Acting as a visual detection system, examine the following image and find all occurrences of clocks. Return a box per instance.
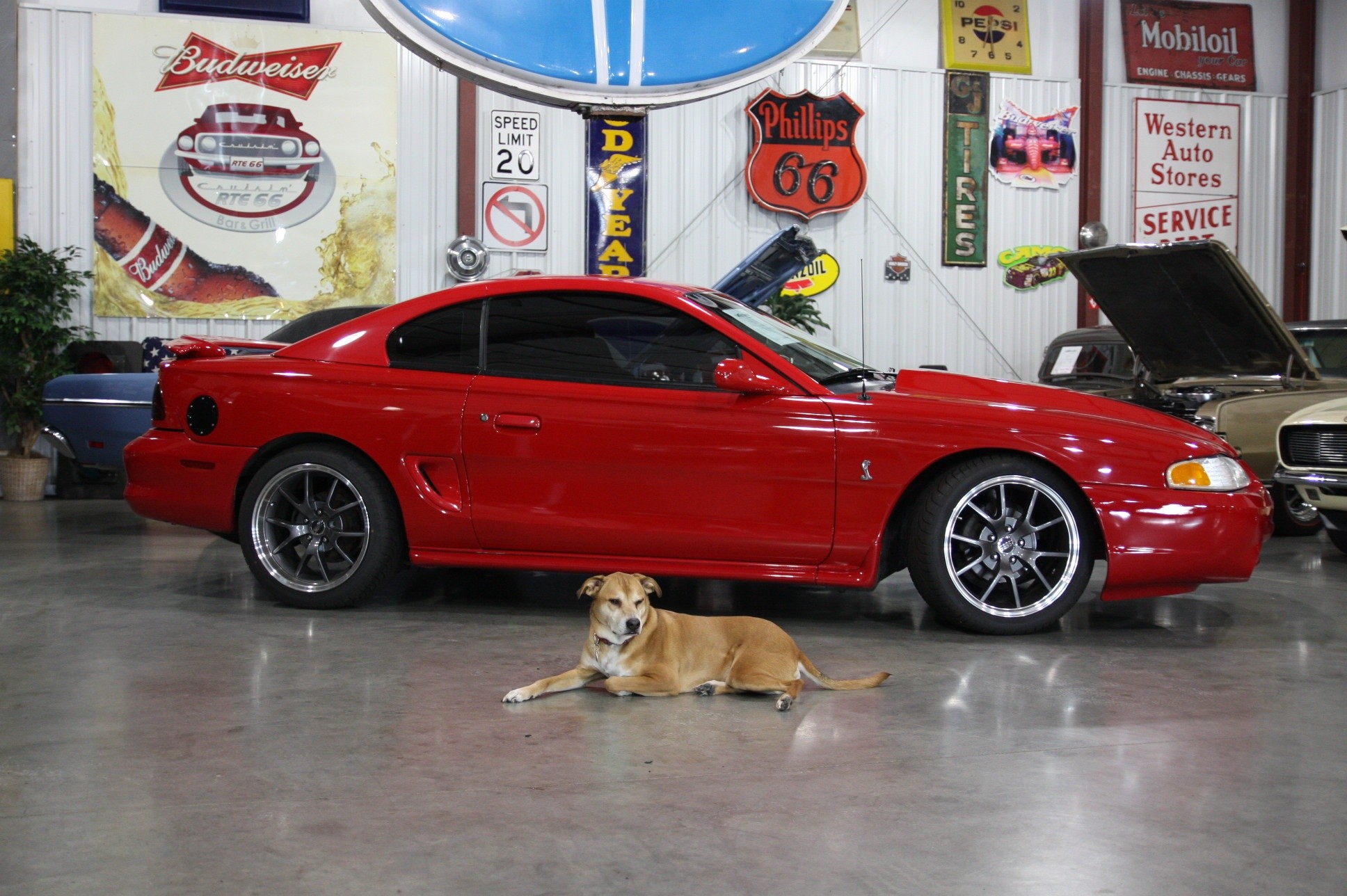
[942,0,1033,76]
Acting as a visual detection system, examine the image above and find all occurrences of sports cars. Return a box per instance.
[1002,256,1068,289]
[124,278,1271,634]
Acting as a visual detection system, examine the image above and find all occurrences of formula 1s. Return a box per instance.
[990,122,1076,175]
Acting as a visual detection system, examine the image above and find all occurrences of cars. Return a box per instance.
[1053,241,1347,539]
[1276,393,1347,556]
[32,304,402,496]
[176,104,323,182]
[1030,327,1162,404]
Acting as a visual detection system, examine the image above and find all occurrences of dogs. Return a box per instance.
[501,570,892,714]
